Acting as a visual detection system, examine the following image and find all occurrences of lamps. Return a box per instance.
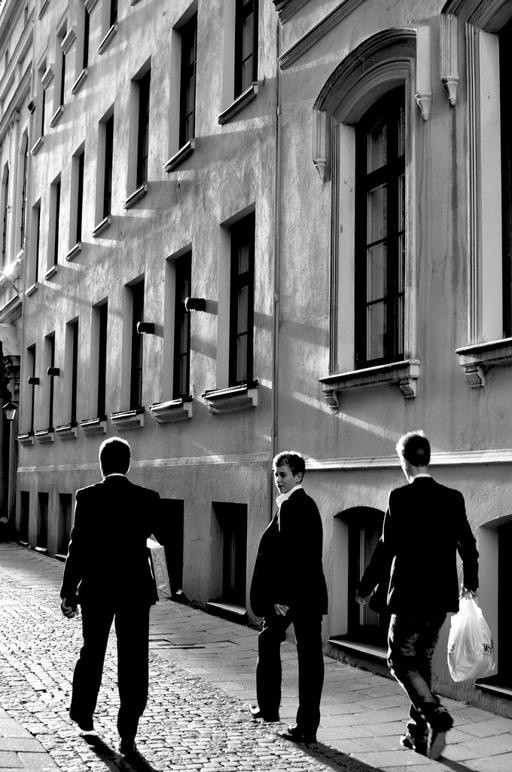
[185,297,206,311]
[48,367,59,376]
[28,377,39,384]
[137,322,155,333]
[2,401,18,421]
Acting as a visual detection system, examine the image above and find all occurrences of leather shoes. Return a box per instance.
[286,722,318,743]
[243,703,280,722]
[118,737,139,755]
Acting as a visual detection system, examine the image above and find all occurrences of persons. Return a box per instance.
[59,436,173,754]
[354,433,480,758]
[248,450,329,744]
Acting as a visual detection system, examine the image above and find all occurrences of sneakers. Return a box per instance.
[400,706,454,760]
